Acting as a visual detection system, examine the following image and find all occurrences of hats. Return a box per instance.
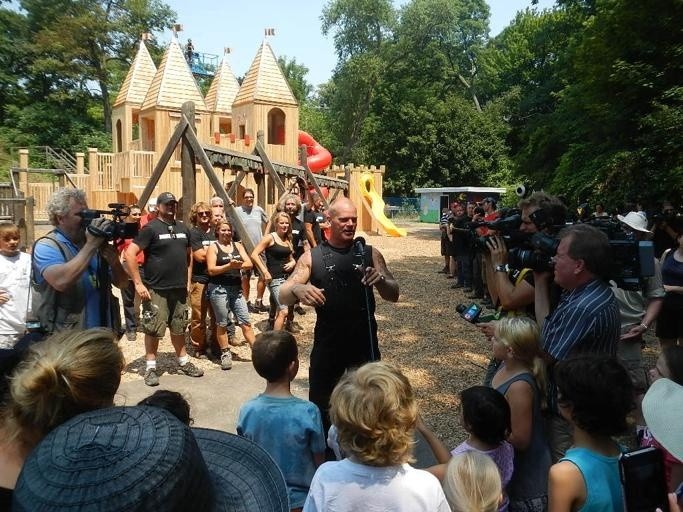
[641,377,683,462]
[616,210,653,236]
[156,192,180,207]
[8,405,292,511]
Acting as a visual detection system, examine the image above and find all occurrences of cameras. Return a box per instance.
[617,446,670,512]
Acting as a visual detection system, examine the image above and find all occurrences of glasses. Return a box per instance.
[198,211,211,219]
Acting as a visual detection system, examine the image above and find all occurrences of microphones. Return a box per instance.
[456,302,484,324]
[354,236,366,264]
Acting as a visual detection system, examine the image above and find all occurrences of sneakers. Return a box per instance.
[255,303,270,312]
[437,268,448,274]
[479,299,493,303]
[463,288,473,293]
[485,302,495,310]
[294,305,319,317]
[220,351,235,372]
[175,360,205,379]
[464,290,477,298]
[450,282,463,290]
[144,368,160,386]
[246,302,260,313]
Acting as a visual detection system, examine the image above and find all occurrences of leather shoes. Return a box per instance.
[126,327,141,342]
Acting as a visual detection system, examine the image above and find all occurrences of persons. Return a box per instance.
[235,328,326,512]
[264,193,306,317]
[326,414,346,460]
[210,196,225,212]
[443,449,504,512]
[302,361,454,512]
[303,193,325,248]
[541,224,622,464]
[29,184,129,335]
[421,386,514,511]
[278,198,399,460]
[206,219,257,371]
[639,343,682,494]
[231,188,271,313]
[482,317,553,511]
[0,324,127,512]
[124,191,204,387]
[137,389,195,429]
[114,204,149,342]
[0,221,40,400]
[570,192,682,228]
[439,192,570,325]
[656,229,683,385]
[642,378,683,511]
[250,211,298,330]
[187,201,218,359]
[611,211,667,394]
[546,350,636,511]
[211,208,224,240]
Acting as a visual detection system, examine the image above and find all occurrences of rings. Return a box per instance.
[380,276,384,280]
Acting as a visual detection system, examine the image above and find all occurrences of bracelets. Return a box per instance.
[291,285,298,300]
[641,324,649,329]
